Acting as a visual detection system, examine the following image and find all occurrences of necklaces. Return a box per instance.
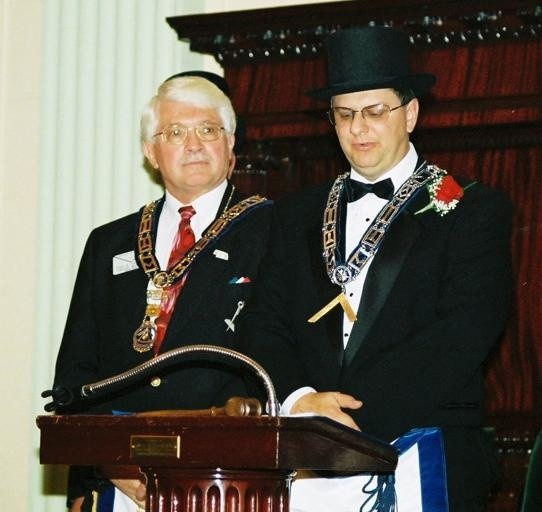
[134,194,267,353]
[307,164,444,322]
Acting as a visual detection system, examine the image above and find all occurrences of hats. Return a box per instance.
[303,26,436,101]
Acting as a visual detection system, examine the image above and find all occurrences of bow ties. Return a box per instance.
[343,178,395,202]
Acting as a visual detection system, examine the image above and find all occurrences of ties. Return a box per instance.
[151,205,197,357]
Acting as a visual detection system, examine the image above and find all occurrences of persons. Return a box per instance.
[226,30,515,511]
[49,75,295,511]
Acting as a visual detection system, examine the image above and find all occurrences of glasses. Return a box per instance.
[153,119,225,145]
[326,103,405,126]
